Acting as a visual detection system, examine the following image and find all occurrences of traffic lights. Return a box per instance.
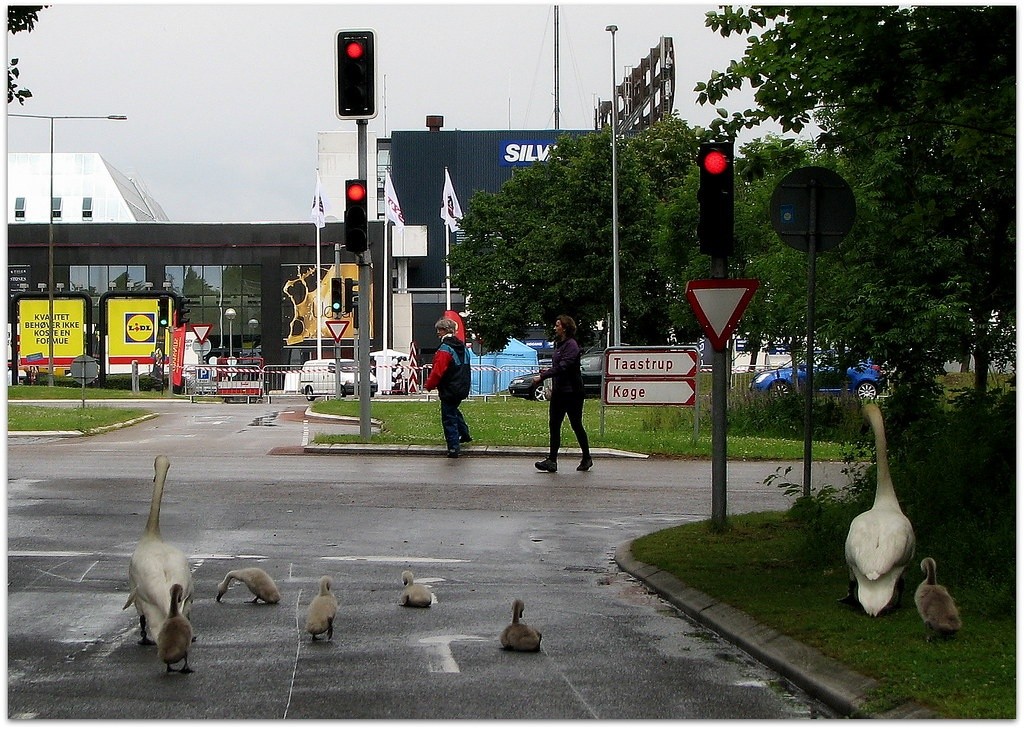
[334,29,378,119]
[344,179,368,253]
[696,141,734,257]
[331,278,341,312]
[345,278,358,312]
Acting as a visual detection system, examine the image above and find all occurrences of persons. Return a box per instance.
[426,318,472,459]
[533,316,594,472]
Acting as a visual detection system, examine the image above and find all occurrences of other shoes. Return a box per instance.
[447,448,458,458]
[575,456,594,471]
[535,456,558,472]
[459,434,472,443]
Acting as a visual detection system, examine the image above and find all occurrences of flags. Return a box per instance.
[311,172,325,228]
[440,170,463,233]
[385,172,404,226]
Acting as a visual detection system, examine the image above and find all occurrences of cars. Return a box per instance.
[509,351,603,402]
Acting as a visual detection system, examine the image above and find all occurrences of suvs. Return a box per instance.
[748,350,885,402]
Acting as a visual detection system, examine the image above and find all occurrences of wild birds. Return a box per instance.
[122,455,194,648]
[844,404,916,618]
[215,567,282,606]
[306,576,338,644]
[500,600,543,652]
[157,584,193,673]
[914,557,963,643]
[399,571,434,607]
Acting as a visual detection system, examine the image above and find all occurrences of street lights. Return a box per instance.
[8,114,128,386]
[605,24,623,382]
[247,319,259,367]
[225,308,237,357]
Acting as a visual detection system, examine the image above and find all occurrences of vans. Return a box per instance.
[300,358,378,402]
